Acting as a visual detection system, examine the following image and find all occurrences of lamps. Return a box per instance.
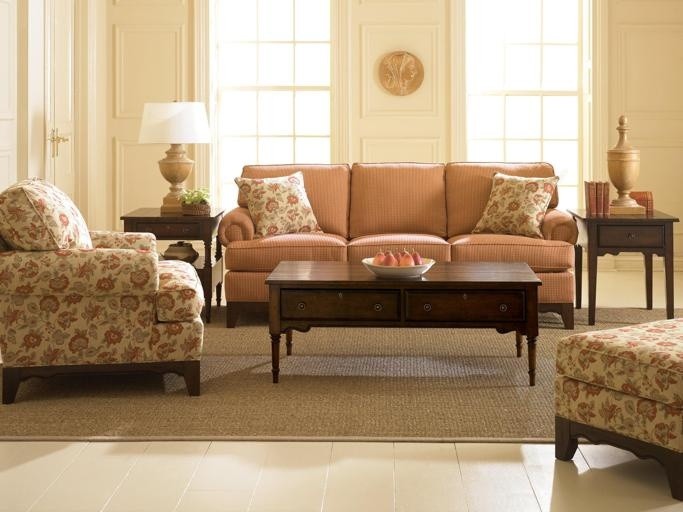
[138,100,216,214]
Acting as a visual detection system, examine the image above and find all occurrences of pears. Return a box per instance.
[373,246,422,267]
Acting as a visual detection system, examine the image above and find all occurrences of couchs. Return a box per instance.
[218,163,578,330]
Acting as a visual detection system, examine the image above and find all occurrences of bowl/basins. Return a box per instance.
[361,257,436,279]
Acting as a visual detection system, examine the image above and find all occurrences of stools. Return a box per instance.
[553,319,683,499]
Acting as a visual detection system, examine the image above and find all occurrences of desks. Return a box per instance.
[119,204,224,323]
[564,209,679,325]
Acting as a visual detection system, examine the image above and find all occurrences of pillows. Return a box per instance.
[470,169,559,239]
[232,170,323,238]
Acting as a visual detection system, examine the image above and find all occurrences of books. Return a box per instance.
[602,182,611,218]
[584,180,596,218]
[595,181,604,219]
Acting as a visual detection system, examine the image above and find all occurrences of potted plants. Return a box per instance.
[177,185,213,218]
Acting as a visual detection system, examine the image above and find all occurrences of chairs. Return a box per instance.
[0,178,202,406]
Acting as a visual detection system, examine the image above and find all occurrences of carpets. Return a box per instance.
[0,307,682,444]
